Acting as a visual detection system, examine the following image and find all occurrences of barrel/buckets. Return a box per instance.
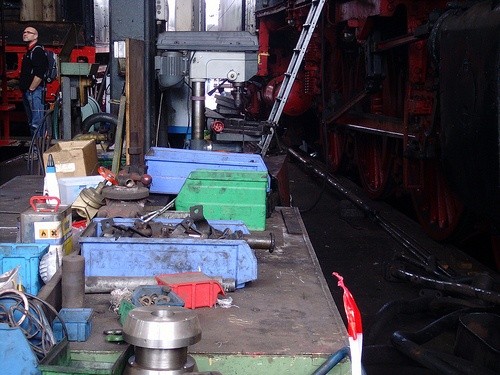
[20,195,72,270]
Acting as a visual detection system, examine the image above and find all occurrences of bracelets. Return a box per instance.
[29,88,33,91]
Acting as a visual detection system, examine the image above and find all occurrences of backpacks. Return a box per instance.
[30,46,60,83]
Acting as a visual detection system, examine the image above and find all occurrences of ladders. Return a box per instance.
[257,0,326,156]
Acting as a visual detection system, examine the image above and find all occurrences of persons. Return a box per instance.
[19,27,49,160]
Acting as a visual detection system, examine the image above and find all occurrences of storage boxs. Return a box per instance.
[42,138,98,177]
[53,308,95,342]
[145,147,270,231]
[0,242,50,297]
[58,174,106,205]
[156,272,225,309]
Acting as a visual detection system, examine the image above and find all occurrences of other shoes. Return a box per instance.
[24,151,39,160]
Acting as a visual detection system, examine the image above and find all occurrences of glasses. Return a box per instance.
[23,31,36,35]
